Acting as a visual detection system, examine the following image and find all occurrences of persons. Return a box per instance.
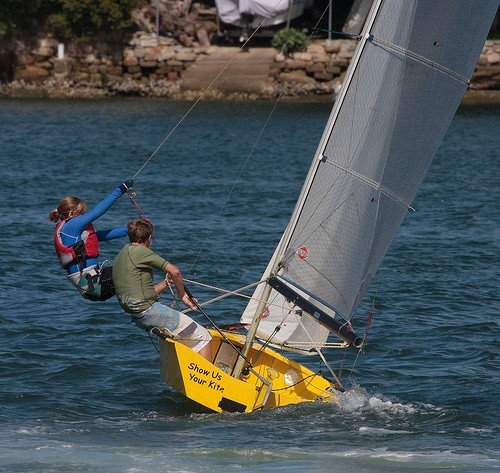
[49,178,134,301]
[111,217,213,362]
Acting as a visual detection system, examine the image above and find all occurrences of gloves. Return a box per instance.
[119,179,134,193]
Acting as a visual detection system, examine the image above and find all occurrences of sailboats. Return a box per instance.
[146,0,500,418]
